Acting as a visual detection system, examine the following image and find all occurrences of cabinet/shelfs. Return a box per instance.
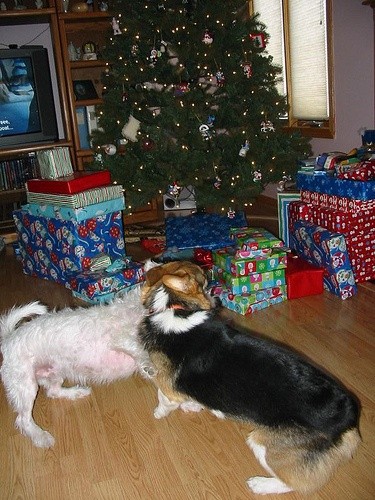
[0,1,167,248]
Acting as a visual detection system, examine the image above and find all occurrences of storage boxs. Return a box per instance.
[13,147,375,315]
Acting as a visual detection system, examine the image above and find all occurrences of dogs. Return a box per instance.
[0,259,217,448]
[138,284,363,495]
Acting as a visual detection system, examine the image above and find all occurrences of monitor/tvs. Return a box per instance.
[0,48,59,149]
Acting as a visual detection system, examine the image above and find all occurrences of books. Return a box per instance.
[0,155,36,218]
[76,105,105,150]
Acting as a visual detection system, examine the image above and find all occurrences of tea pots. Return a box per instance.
[68,41,78,61]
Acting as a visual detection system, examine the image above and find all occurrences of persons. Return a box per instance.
[75,83,86,96]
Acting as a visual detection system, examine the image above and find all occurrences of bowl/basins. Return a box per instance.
[83,53,97,61]
